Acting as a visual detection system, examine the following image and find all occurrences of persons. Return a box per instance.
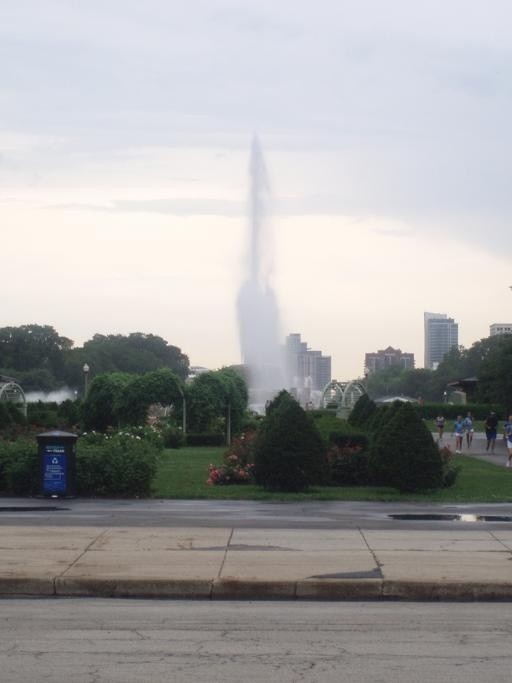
[436,410,512,467]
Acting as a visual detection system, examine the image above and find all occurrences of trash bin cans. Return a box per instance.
[36,431,78,498]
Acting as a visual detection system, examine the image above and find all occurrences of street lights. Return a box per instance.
[83,363,89,399]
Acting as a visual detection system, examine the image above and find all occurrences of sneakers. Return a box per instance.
[455,450,462,454]
[505,461,512,467]
